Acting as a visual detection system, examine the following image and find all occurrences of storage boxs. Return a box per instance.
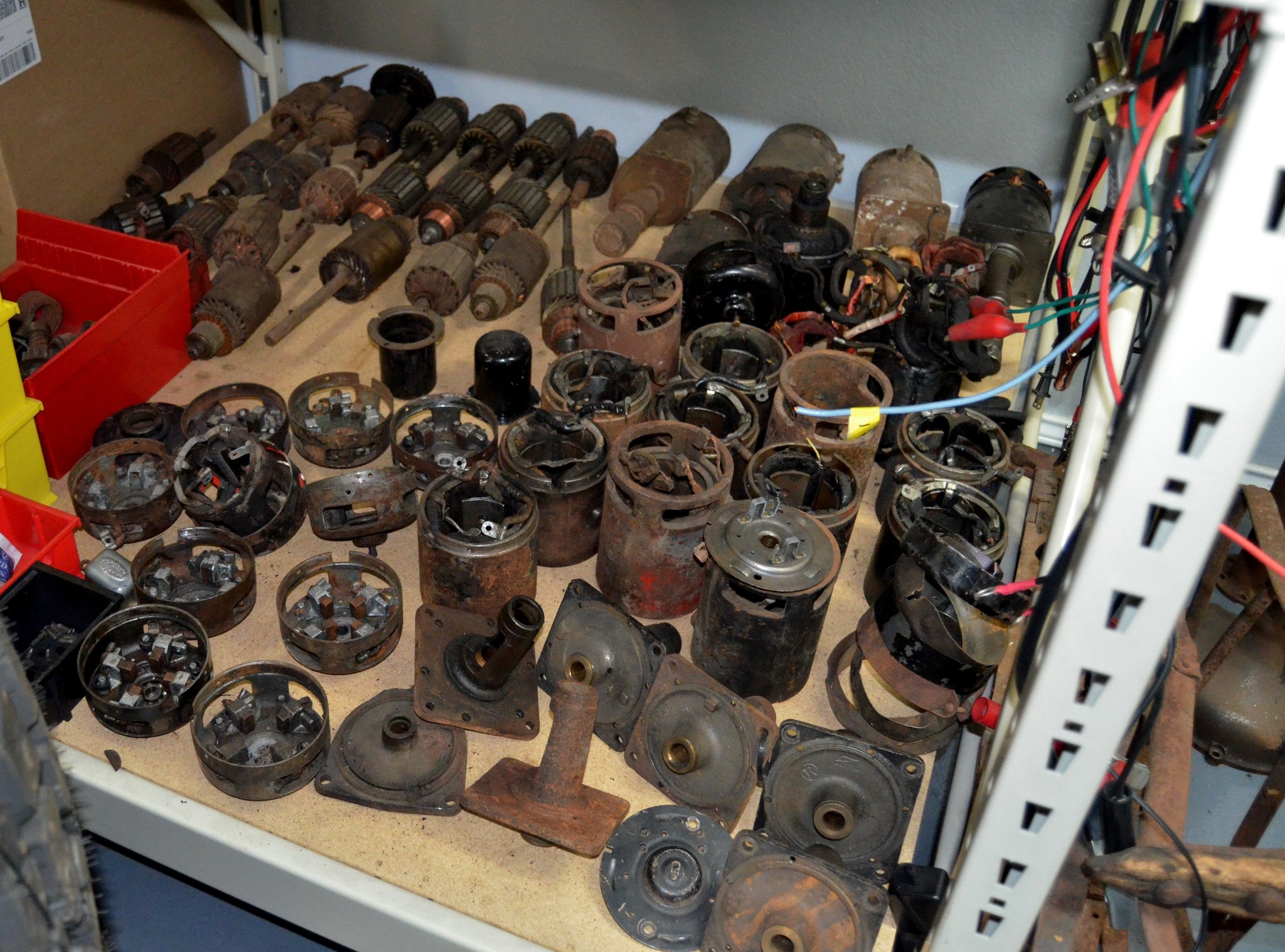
[0,487,86,594]
[0,561,124,730]
[0,209,195,478]
